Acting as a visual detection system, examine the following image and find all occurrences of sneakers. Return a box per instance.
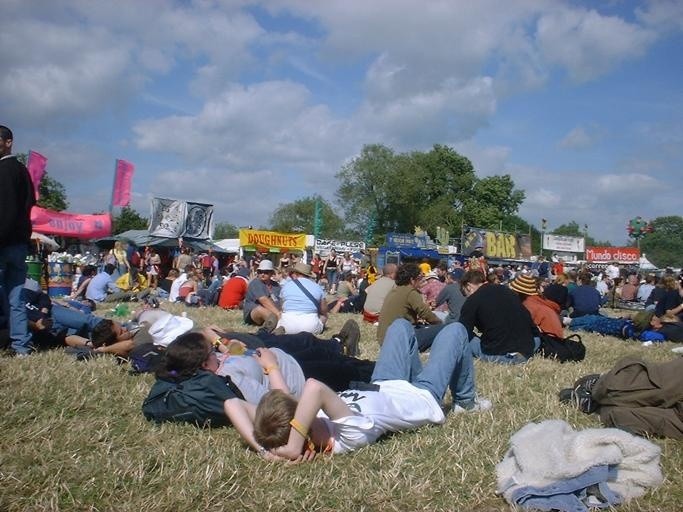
[344,322,361,357]
[332,318,354,343]
[271,326,285,335]
[451,399,493,414]
[255,313,278,334]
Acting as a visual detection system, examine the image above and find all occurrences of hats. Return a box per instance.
[287,262,317,278]
[148,314,194,348]
[508,272,540,296]
[236,268,251,281]
[633,308,656,331]
[257,260,275,272]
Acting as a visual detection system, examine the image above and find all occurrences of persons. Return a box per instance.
[377,264,449,352]
[427,268,471,323]
[163,270,179,292]
[561,310,677,350]
[596,275,610,308]
[521,264,528,274]
[532,256,544,273]
[359,249,369,270]
[147,245,161,290]
[476,256,489,282]
[244,259,282,325]
[417,272,448,311]
[657,277,683,315]
[619,264,630,276]
[622,275,637,303]
[457,270,536,366]
[334,270,357,313]
[218,269,248,308]
[20,263,144,348]
[553,257,565,275]
[508,274,565,339]
[443,271,456,284]
[356,266,378,310]
[565,271,578,284]
[86,264,137,302]
[148,332,378,403]
[630,309,683,344]
[199,250,212,283]
[339,251,360,282]
[221,318,492,465]
[609,277,631,310]
[666,263,673,276]
[176,246,192,269]
[50,298,96,316]
[276,253,290,269]
[417,256,431,275]
[219,318,363,351]
[363,263,396,324]
[435,263,448,278]
[495,267,505,282]
[109,241,132,276]
[186,280,220,307]
[605,260,620,285]
[322,249,339,295]
[568,272,602,317]
[310,254,324,282]
[637,275,656,306]
[557,355,682,440]
[169,264,195,302]
[70,265,97,300]
[469,246,482,260]
[65,319,285,354]
[179,269,204,304]
[0,124,37,357]
[276,262,329,335]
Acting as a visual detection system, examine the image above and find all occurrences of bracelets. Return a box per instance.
[213,341,225,350]
[84,340,93,348]
[263,364,282,376]
[289,421,311,443]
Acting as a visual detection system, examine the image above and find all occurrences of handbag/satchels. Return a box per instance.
[537,331,586,363]
